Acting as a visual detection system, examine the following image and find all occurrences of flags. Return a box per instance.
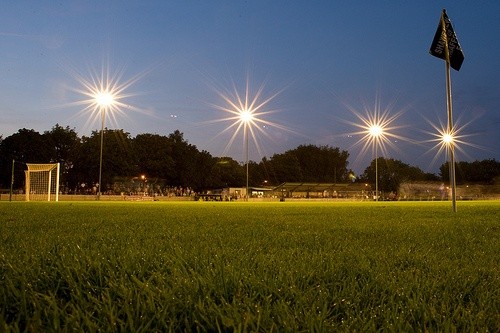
[429,9,465,72]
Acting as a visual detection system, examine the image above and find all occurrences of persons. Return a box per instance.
[58,181,463,203]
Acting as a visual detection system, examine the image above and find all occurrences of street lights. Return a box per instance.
[94,90,112,200]
[442,133,454,199]
[239,109,254,201]
[368,124,383,202]
